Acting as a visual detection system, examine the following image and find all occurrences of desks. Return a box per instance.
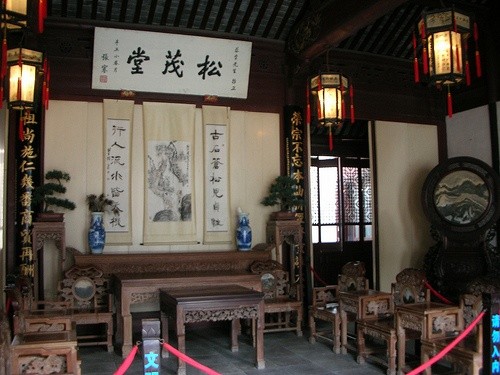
[113,269,262,359]
[159,284,265,375]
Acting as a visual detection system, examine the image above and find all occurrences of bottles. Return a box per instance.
[235,213,253,251]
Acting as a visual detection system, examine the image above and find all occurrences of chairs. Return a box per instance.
[0,308,82,375]
[308,261,369,354]
[421,276,500,375]
[247,259,303,337]
[357,268,431,375]
[14,279,72,334]
[57,266,116,353]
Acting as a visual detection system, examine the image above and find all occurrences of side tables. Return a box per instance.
[339,289,391,362]
[395,301,459,375]
[11,330,78,357]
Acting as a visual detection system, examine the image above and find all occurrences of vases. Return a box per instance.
[88,212,106,255]
[235,212,252,251]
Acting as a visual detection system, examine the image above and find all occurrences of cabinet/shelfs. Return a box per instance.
[266,220,303,328]
[33,222,65,305]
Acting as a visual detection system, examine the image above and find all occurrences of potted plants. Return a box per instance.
[260,176,305,220]
[33,169,76,222]
